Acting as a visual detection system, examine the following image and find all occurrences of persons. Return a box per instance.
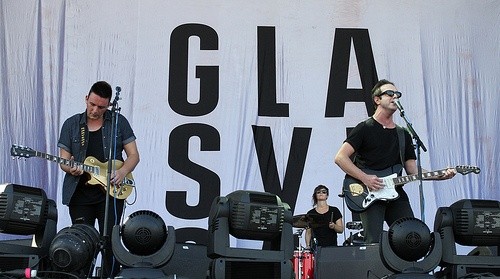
[334,78,456,246]
[305,184,343,250]
[57,81,141,279]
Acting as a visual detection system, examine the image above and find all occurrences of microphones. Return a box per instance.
[394,99,404,113]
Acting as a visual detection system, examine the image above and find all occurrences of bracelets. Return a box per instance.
[306,244,310,246]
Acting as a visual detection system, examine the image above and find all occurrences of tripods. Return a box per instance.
[88,86,122,279]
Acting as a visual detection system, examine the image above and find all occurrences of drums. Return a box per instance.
[293,250,315,279]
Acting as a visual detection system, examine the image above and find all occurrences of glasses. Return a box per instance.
[377,91,403,98]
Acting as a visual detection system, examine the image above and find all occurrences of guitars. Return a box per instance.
[340,165,481,213]
[10,143,134,200]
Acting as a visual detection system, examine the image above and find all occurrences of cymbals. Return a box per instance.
[292,213,331,229]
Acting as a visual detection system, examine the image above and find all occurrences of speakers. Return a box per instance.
[160,242,215,279]
[316,244,395,279]
